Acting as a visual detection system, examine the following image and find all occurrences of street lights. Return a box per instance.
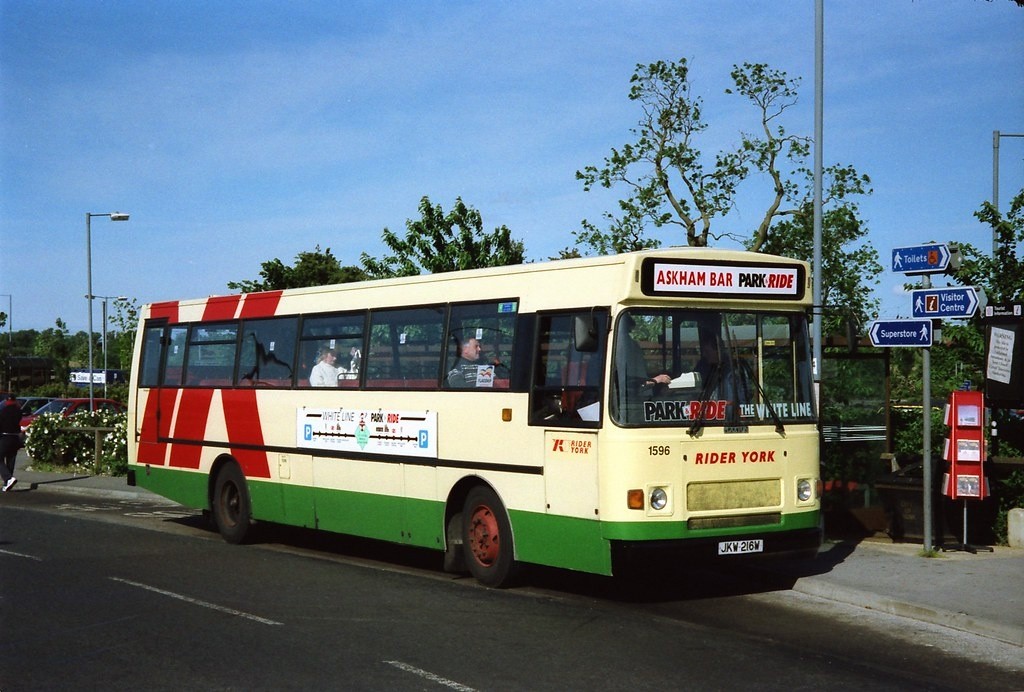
[85,212,130,412]
[85,293,127,398]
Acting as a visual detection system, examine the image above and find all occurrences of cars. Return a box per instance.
[19,398,128,438]
[16,396,53,418]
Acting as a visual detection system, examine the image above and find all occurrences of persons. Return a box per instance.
[307,342,361,387]
[0,392,23,492]
[693,333,752,388]
[448,335,500,390]
[617,311,672,400]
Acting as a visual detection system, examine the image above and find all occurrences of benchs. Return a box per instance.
[165,360,602,412]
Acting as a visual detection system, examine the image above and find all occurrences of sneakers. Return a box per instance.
[2,477,17,492]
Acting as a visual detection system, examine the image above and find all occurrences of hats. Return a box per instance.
[9,393,16,398]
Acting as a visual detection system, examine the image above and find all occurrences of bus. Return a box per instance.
[128,247,824,601]
